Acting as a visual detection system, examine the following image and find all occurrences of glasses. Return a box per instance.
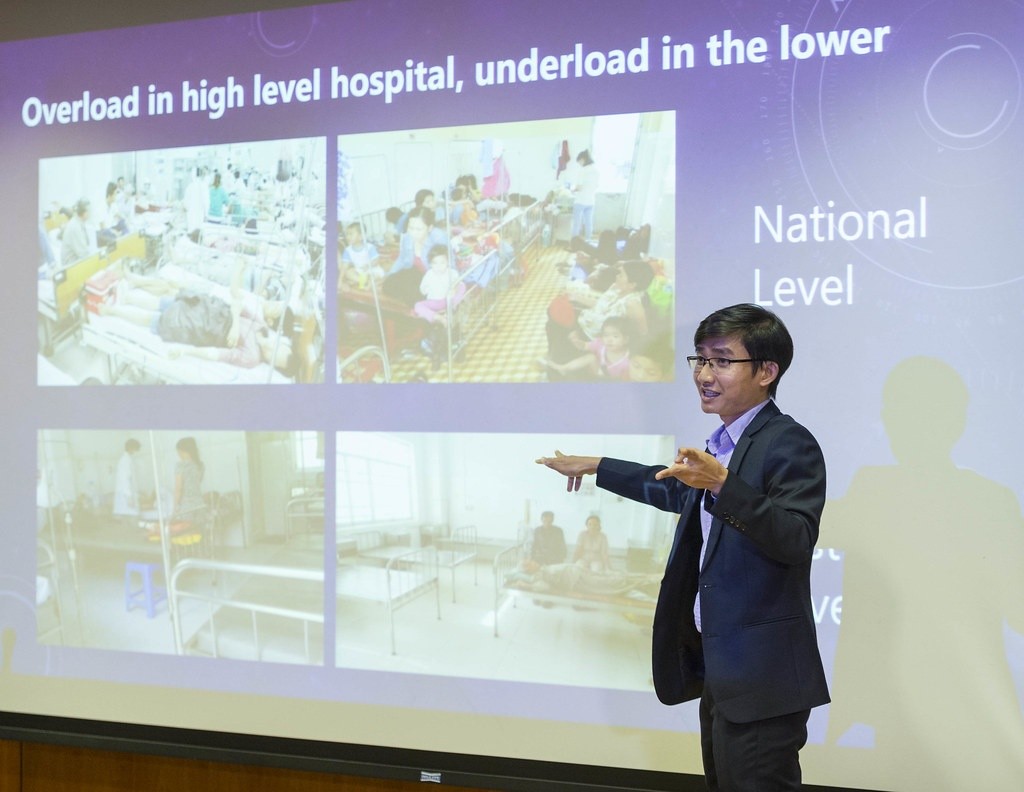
[687,356,773,373]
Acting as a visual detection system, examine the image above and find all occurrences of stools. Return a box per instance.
[122,560,181,617]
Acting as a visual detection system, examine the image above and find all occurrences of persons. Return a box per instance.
[526,512,566,563]
[173,437,207,518]
[338,152,537,325]
[39,157,307,367]
[574,516,611,573]
[569,151,602,242]
[115,437,144,516]
[539,223,669,378]
[536,302,828,792]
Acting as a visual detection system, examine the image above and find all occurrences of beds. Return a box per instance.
[345,525,663,659]
[169,494,325,665]
[59,506,222,599]
[336,192,544,383]
[39,203,325,383]
[215,489,250,554]
